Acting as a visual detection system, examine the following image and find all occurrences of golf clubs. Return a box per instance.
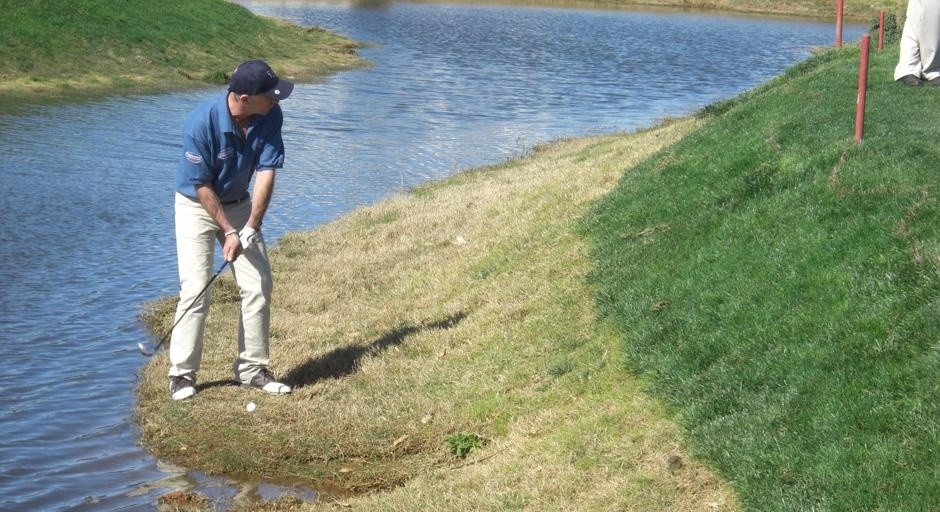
[136,241,241,357]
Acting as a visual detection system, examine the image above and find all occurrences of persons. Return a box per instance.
[166,58,293,401]
[893,0,939,87]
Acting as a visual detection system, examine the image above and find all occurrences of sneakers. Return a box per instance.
[169,376,197,400]
[250,369,291,394]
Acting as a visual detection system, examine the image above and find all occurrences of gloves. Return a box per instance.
[238,226,259,254]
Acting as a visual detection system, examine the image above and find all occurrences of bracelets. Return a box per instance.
[224,228,237,237]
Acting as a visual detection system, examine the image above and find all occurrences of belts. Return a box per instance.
[221,192,249,205]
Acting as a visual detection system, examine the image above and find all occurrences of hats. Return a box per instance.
[230,60,294,100]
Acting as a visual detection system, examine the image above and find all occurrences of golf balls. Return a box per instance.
[245,402,257,413]
